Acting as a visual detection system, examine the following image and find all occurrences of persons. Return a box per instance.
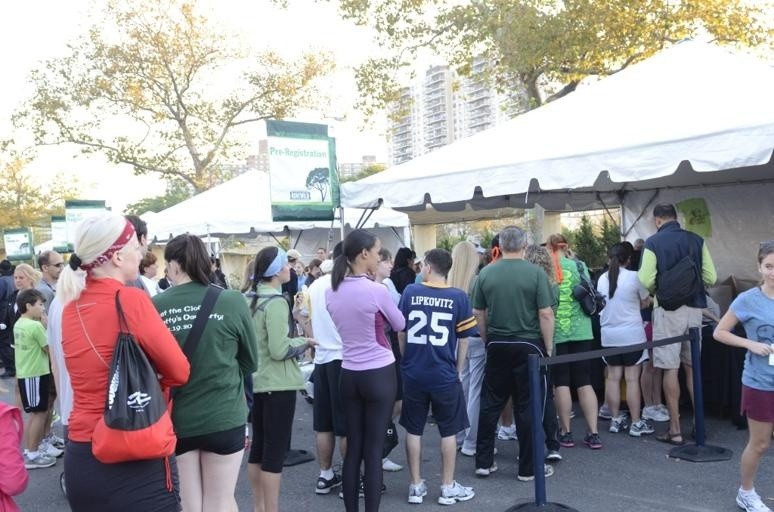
[712,242,774,512]
[0,201,721,511]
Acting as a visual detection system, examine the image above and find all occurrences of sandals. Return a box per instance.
[655,430,685,444]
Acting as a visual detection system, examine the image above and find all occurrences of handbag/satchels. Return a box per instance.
[102,335,167,430]
[656,257,703,312]
[573,279,606,317]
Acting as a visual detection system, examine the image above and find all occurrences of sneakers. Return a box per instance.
[556,412,575,419]
[609,416,628,434]
[642,406,671,421]
[516,464,554,481]
[37,440,64,457]
[629,421,655,436]
[476,462,499,475]
[545,449,562,459]
[50,434,65,448]
[338,482,387,499]
[299,383,314,404]
[557,430,575,448]
[498,425,519,441]
[460,445,499,456]
[381,456,403,472]
[656,404,681,418]
[50,412,61,428]
[583,432,603,450]
[315,470,342,494]
[21,451,56,470]
[735,486,773,512]
[599,405,628,419]
[436,480,475,506]
[405,478,429,504]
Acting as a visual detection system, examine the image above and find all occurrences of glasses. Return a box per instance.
[758,238,773,248]
[46,261,65,268]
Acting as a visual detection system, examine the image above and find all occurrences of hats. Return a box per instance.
[286,249,301,258]
